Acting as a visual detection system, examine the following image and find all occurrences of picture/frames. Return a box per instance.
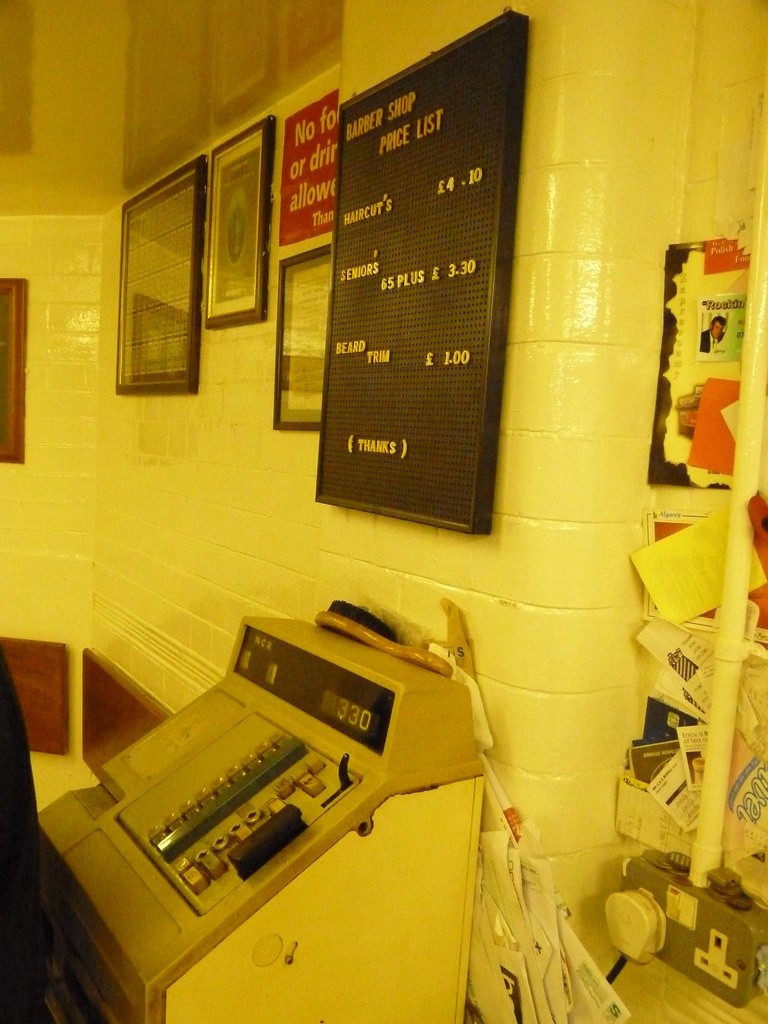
[115,155,208,395]
